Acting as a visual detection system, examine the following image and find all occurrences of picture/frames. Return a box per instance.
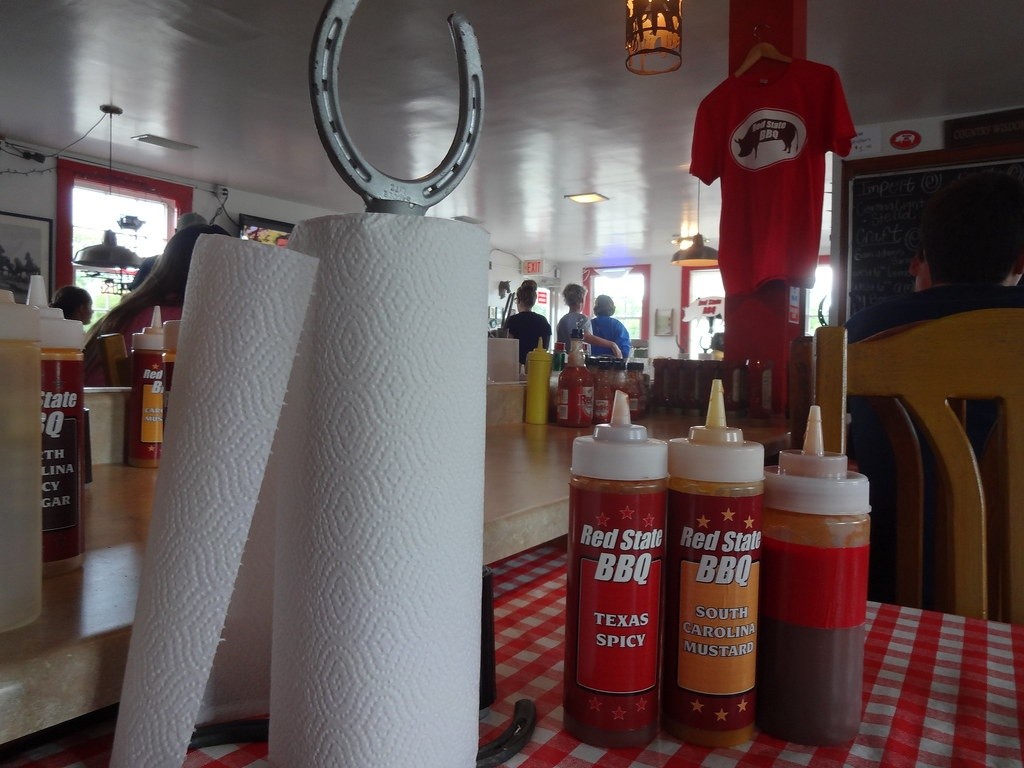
[0,211,54,305]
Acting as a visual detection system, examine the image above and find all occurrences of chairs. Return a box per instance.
[789,308,1024,626]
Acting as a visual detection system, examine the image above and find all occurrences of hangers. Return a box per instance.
[733,23,791,78]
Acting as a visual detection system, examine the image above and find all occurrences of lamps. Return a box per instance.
[625,0,683,75]
[671,178,718,266]
[71,104,143,268]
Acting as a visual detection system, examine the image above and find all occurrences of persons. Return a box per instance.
[275,236,288,247]
[127,212,208,290]
[710,333,724,357]
[48,285,93,326]
[503,280,552,373]
[590,295,631,358]
[82,224,230,387]
[557,284,622,358]
[846,174,1024,601]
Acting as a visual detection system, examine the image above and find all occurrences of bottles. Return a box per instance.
[0,289,43,634]
[26,274,86,579]
[524,328,650,428]
[658,379,766,751]
[126,305,182,469]
[562,390,668,751]
[753,405,872,749]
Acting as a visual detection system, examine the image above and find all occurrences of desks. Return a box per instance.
[0,527,1024,768]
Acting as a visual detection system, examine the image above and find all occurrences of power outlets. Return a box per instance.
[218,186,230,198]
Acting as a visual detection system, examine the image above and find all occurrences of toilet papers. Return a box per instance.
[108,214,492,766]
